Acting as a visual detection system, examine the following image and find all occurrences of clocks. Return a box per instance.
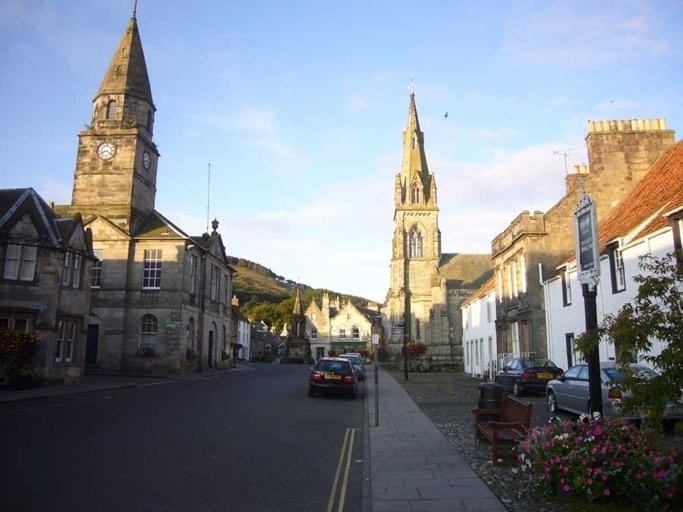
[97,141,117,160]
[143,151,151,170]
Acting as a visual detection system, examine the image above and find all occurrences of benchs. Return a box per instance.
[472,391,533,466]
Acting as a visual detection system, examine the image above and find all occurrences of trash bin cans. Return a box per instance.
[478,383,506,443]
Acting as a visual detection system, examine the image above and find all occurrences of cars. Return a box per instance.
[494,357,683,424]
[308,353,365,397]
